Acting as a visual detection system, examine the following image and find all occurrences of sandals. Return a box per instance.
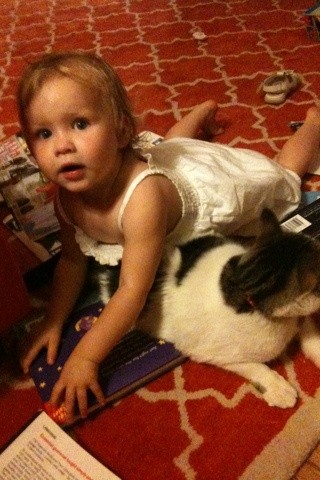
[261,70,304,104]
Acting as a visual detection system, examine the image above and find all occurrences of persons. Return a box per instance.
[16,52,320,421]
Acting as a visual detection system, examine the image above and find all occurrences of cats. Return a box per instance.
[90,206,320,410]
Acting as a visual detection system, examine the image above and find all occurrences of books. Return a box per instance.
[0,127,190,480]
[278,199,320,245]
